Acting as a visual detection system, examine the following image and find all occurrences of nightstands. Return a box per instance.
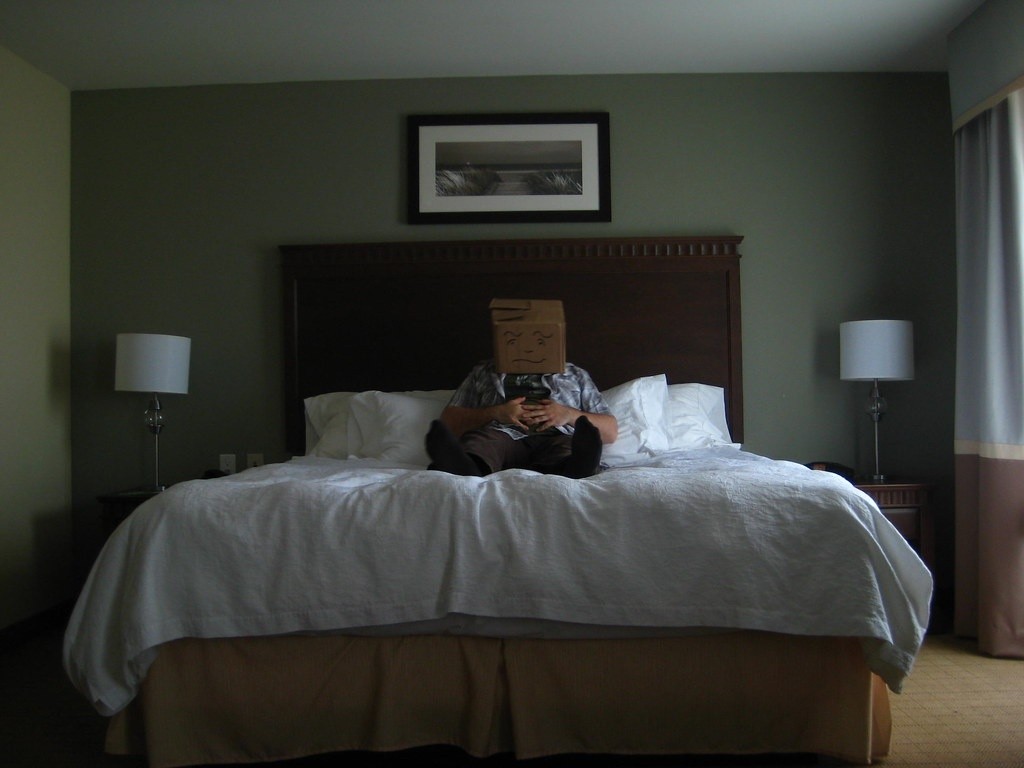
[851,482,943,634]
[73,486,164,599]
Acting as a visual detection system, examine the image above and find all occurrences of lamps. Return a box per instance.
[113,332,192,495]
[838,318,917,483]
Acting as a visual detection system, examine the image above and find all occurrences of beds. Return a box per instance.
[60,233,935,767]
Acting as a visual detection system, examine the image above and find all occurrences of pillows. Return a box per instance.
[600,374,670,468]
[303,387,458,460]
[668,383,741,453]
[350,391,442,464]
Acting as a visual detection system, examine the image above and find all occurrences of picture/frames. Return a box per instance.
[402,111,613,224]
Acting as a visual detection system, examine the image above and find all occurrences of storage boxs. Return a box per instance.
[489,297,564,374]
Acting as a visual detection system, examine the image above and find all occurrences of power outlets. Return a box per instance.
[218,452,237,478]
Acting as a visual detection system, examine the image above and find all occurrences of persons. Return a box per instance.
[427,362,615,479]
